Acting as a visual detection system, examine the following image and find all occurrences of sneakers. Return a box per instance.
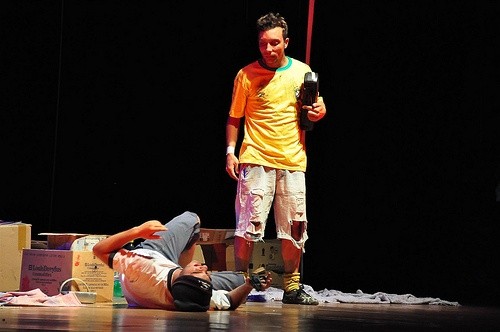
[282,285,319,305]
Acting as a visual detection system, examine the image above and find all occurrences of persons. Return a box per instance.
[225,13,326,304]
[93,211,273,311]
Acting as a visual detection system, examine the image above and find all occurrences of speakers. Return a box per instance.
[199,227,236,271]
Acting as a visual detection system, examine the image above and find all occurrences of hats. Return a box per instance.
[170,274,213,313]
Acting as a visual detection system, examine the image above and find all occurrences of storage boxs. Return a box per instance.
[19,246,114,303]
[226,238,284,287]
[0,223,31,291]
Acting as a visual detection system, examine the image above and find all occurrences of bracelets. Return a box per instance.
[226,146,234,155]
